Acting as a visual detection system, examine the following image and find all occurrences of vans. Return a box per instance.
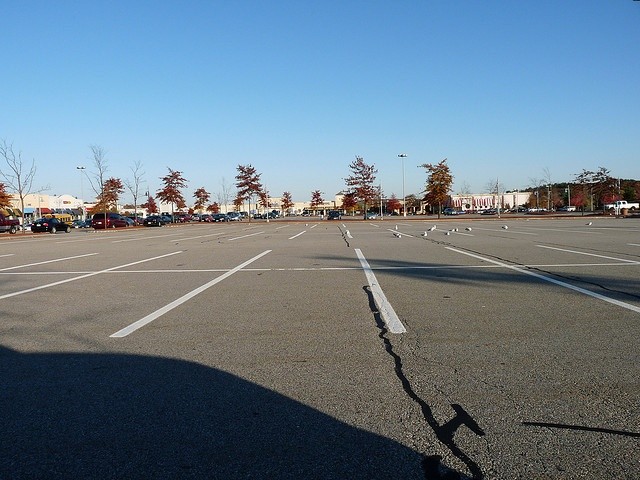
[0,208,20,234]
[564,206,576,212]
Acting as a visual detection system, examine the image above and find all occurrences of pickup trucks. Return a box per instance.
[605,200,639,211]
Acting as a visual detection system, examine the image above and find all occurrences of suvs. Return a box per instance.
[327,211,342,220]
[91,213,129,228]
[443,208,456,216]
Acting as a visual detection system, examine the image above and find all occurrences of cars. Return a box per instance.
[212,214,230,222]
[199,214,213,223]
[31,218,71,233]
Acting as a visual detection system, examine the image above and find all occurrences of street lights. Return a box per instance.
[398,153,407,217]
[77,167,86,221]
[145,191,151,217]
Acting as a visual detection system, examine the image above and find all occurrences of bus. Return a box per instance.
[250,209,256,217]
[42,214,73,227]
